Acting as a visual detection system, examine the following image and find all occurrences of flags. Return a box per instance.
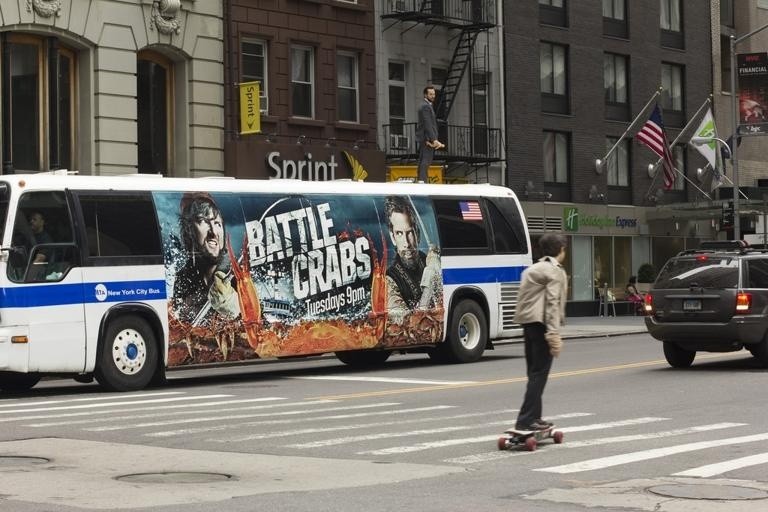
[712,137,740,189]
[458,200,484,221]
[689,109,720,172]
[636,105,675,189]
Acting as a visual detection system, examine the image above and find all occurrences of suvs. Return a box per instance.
[644,239,768,370]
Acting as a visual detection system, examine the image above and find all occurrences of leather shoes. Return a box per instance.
[516,422,549,431]
[536,419,553,426]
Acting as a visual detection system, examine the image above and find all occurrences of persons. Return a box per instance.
[378,193,442,327]
[413,84,446,184]
[170,190,248,331]
[625,277,651,314]
[25,212,60,260]
[515,229,568,428]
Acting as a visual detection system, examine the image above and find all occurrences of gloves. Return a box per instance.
[420,244,440,293]
[207,271,242,323]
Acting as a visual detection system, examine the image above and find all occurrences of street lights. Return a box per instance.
[690,135,740,241]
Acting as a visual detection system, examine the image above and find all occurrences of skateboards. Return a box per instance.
[499,423,564,450]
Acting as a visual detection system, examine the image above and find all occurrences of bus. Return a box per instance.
[0,169,533,396]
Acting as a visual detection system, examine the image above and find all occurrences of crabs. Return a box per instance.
[168,230,444,366]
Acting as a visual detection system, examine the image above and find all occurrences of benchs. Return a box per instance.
[598,288,649,316]
